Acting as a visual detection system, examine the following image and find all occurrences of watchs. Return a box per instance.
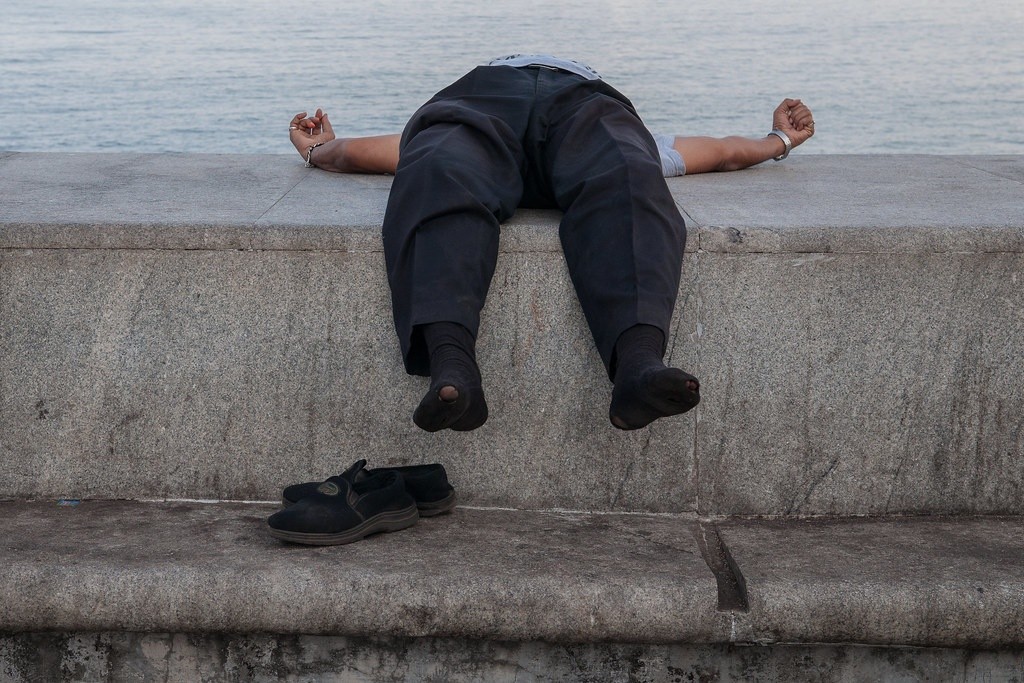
[768,131,791,160]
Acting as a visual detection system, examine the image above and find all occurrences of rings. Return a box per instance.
[289,127,297,130]
[807,121,815,126]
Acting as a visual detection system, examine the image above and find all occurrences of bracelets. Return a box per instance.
[305,142,326,168]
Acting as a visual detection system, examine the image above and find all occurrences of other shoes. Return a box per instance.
[282,460,457,516]
[268,470,422,547]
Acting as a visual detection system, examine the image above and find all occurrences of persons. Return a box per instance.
[290,53,815,431]
